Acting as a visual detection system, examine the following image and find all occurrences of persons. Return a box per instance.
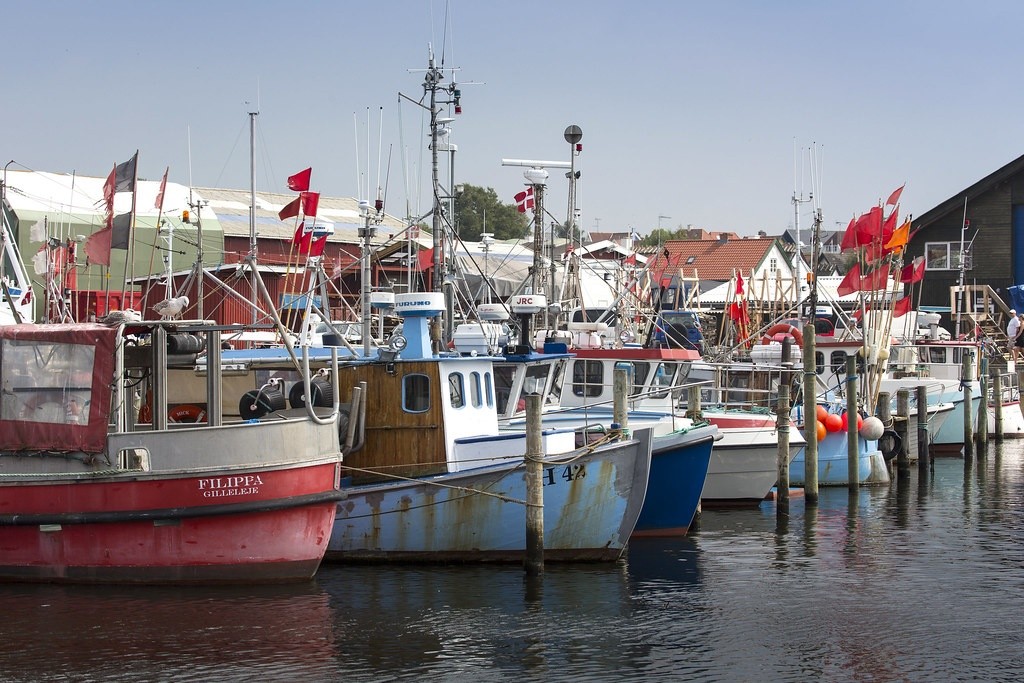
[1006,309,1024,363]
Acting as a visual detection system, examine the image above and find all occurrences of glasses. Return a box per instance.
[1010,313,1013,314]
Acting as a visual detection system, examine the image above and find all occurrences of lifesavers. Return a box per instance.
[877,431,902,461]
[168,404,207,426]
[762,324,803,349]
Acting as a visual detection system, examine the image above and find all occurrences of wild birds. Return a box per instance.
[147,296,190,321]
[96,307,136,327]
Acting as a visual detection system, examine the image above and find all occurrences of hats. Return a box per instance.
[1009,309,1016,314]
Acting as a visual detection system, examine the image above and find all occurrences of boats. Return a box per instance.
[0,42,1023,583]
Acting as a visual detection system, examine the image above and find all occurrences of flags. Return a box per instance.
[279,192,327,256]
[288,168,310,190]
[514,187,534,212]
[418,242,443,271]
[728,273,750,349]
[837,184,925,323]
